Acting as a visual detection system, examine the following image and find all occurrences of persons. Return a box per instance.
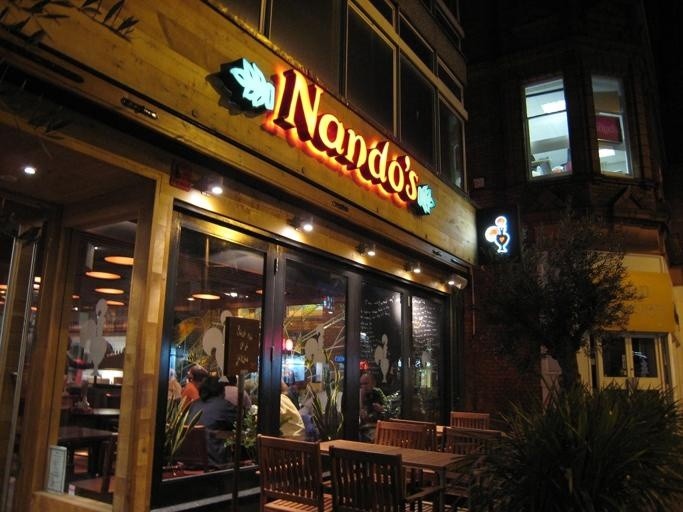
[163,363,391,477]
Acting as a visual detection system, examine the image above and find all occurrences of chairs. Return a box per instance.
[441,425,502,511]
[391,418,437,451]
[256,433,345,511]
[449,411,491,430]
[328,444,452,511]
[373,419,427,510]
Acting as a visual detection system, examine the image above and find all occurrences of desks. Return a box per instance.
[58,428,119,475]
[84,408,120,430]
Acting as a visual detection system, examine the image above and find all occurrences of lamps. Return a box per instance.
[204,175,224,196]
[359,241,375,256]
[407,259,422,273]
[295,215,314,232]
[442,278,455,286]
